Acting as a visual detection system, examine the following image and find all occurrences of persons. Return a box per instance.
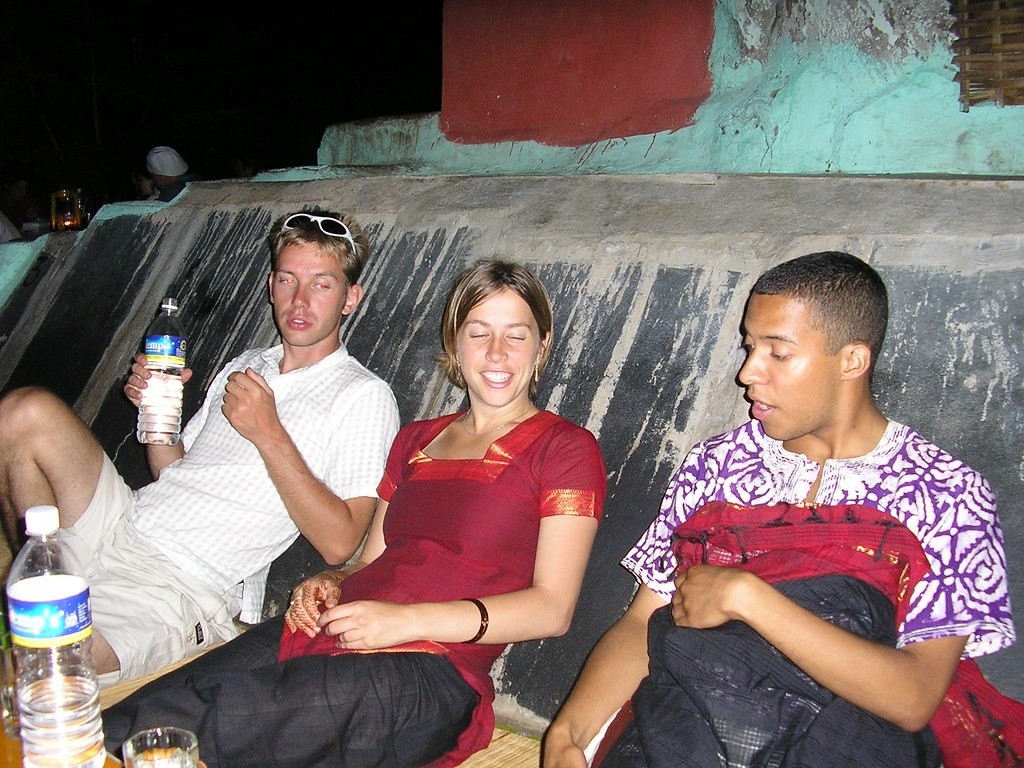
[545,252,1016,768]
[102,261,606,767]
[0,143,266,240]
[0,211,402,697]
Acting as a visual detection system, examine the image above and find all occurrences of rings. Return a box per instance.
[291,596,301,606]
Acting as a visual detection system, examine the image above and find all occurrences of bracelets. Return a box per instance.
[461,598,489,643]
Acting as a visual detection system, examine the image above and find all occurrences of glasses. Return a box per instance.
[273,213,355,256]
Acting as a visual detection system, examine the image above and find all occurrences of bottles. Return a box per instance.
[5,505,106,768]
[136,296,187,447]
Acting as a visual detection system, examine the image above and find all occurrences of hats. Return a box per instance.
[147,146,189,176]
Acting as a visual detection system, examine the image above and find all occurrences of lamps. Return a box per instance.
[50,181,81,231]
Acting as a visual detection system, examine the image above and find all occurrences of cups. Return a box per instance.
[119,725,197,768]
[0,633,22,738]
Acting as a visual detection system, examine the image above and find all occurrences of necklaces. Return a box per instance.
[462,400,533,436]
